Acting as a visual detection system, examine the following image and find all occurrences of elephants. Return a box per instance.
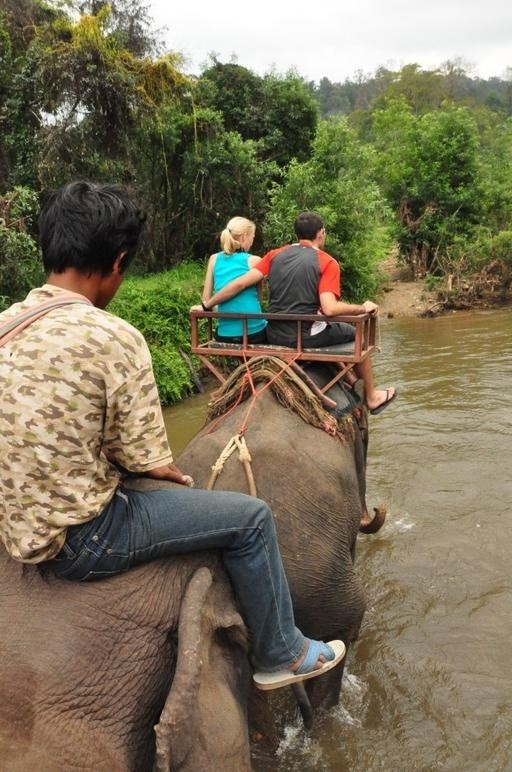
[0,356,388,772]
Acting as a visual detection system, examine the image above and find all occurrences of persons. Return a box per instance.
[0,181,346,690]
[202,215,266,344]
[189,213,397,415]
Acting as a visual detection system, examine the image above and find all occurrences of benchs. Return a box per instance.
[190,303,379,362]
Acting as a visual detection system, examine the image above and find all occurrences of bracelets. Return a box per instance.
[202,303,212,311]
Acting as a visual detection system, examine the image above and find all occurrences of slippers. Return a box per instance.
[371,388,397,416]
[252,640,347,691]
